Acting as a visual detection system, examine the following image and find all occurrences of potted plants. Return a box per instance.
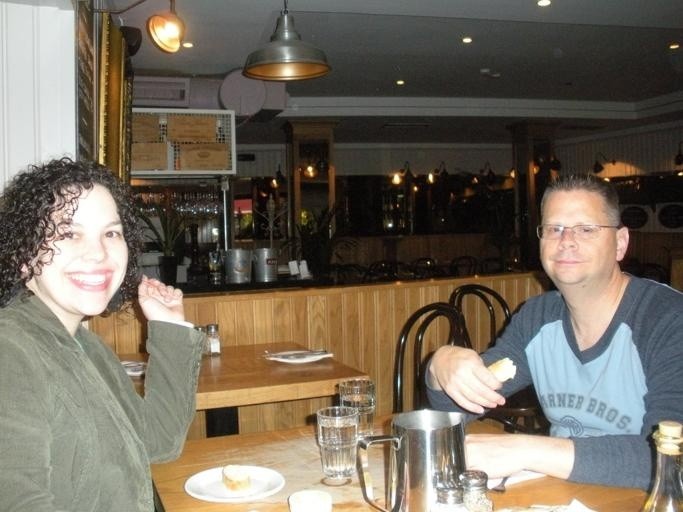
[282,203,355,277]
[136,203,197,285]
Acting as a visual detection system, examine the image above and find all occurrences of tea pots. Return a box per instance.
[355,410,467,512]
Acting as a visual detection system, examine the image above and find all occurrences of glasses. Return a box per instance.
[537,224,618,239]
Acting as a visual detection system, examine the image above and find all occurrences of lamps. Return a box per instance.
[592,152,617,174]
[433,160,449,179]
[482,162,496,178]
[91,0,189,56]
[674,141,682,165]
[398,163,414,179]
[243,0,333,81]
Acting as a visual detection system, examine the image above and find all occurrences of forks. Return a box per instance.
[488,478,511,495]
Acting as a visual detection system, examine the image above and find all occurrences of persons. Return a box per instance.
[1,157,205,512]
[423,171,682,500]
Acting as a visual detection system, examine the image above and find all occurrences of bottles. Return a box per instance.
[193,325,223,356]
[638,419,682,512]
[458,467,494,512]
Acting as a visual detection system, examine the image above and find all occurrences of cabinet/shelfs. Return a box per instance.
[287,119,337,239]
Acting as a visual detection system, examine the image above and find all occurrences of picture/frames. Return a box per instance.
[96,11,131,186]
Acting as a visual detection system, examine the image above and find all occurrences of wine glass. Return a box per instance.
[134,190,222,219]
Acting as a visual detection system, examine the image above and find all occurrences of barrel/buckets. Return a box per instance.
[226,248,252,284]
[255,246,279,283]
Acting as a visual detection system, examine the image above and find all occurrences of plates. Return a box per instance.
[121,360,149,376]
[182,465,283,503]
[267,348,332,365]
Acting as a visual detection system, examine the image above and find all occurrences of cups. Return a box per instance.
[314,407,357,481]
[288,490,331,512]
[207,251,224,285]
[339,379,377,441]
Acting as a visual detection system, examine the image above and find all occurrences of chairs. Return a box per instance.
[441,282,542,435]
[328,252,671,295]
[391,300,462,415]
